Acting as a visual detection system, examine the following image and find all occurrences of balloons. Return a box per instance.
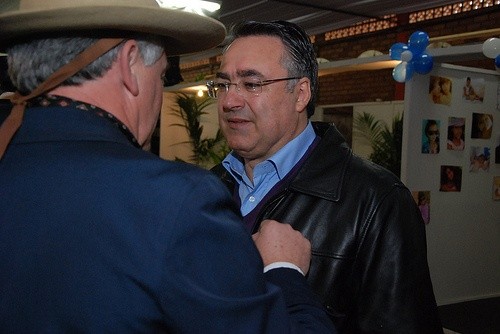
[496,54,500,67]
[482,38,500,59]
[388,31,432,83]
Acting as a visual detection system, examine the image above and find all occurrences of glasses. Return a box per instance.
[205,77,302,100]
[428,130,438,136]
[420,198,425,201]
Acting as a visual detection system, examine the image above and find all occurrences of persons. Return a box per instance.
[418,77,500,224]
[204,22,437,334]
[0,0,311,334]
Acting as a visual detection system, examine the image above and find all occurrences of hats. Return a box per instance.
[0,0,227,57]
